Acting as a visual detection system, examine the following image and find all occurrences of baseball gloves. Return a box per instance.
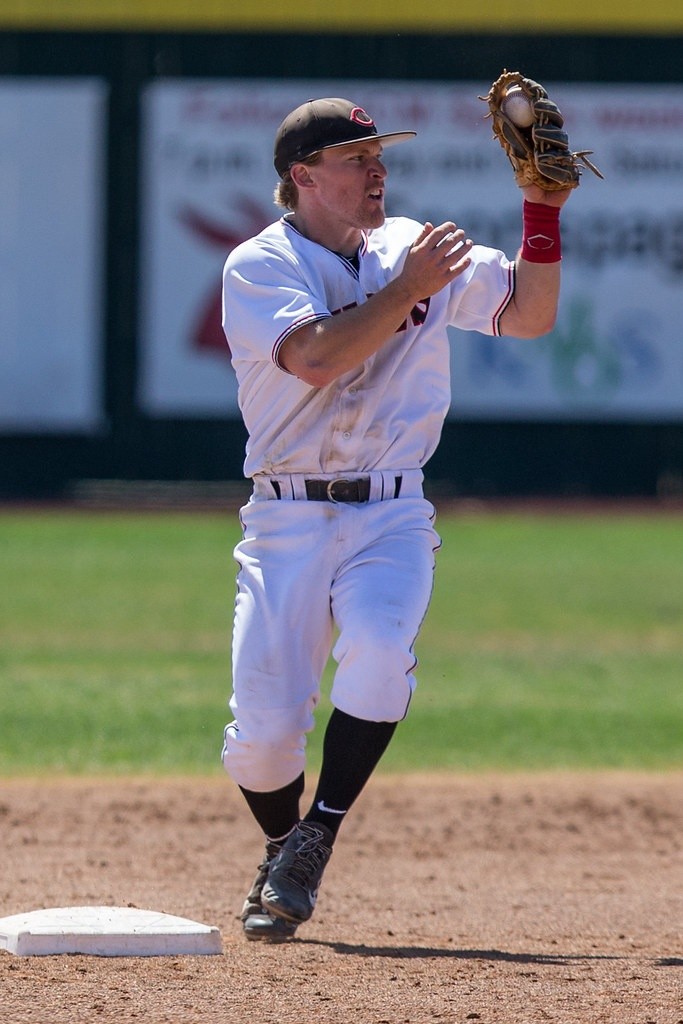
[477,68,607,191]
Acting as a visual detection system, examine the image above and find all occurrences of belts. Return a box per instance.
[269,476,408,504]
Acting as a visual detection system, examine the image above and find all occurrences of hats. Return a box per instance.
[275,98,419,180]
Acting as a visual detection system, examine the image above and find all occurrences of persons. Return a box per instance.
[223,71,604,943]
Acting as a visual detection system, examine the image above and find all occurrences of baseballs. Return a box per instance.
[500,87,534,128]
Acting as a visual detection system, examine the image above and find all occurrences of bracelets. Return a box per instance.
[520,200,561,263]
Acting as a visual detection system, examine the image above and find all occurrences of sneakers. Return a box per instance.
[261,822,332,922]
[242,844,299,941]
